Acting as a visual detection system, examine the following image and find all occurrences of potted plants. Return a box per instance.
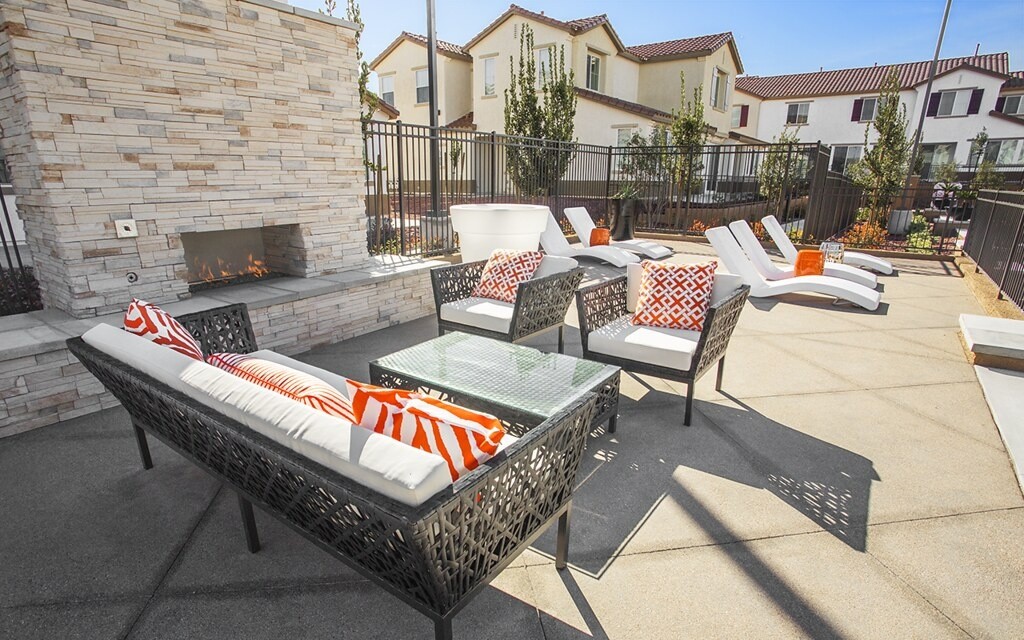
[609,183,643,241]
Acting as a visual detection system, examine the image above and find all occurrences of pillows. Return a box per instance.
[124,298,204,362]
[208,352,356,424]
[344,379,507,503]
[471,249,543,304]
[629,260,719,333]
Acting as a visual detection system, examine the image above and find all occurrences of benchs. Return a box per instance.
[64,324,596,639]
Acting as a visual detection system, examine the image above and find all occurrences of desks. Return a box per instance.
[590,228,610,247]
[794,249,824,277]
[368,331,622,452]
[819,242,844,264]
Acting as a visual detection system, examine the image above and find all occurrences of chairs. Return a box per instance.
[429,253,585,355]
[539,212,640,268]
[564,206,671,259]
[728,220,877,288]
[762,215,892,275]
[705,226,880,310]
[575,263,751,426]
